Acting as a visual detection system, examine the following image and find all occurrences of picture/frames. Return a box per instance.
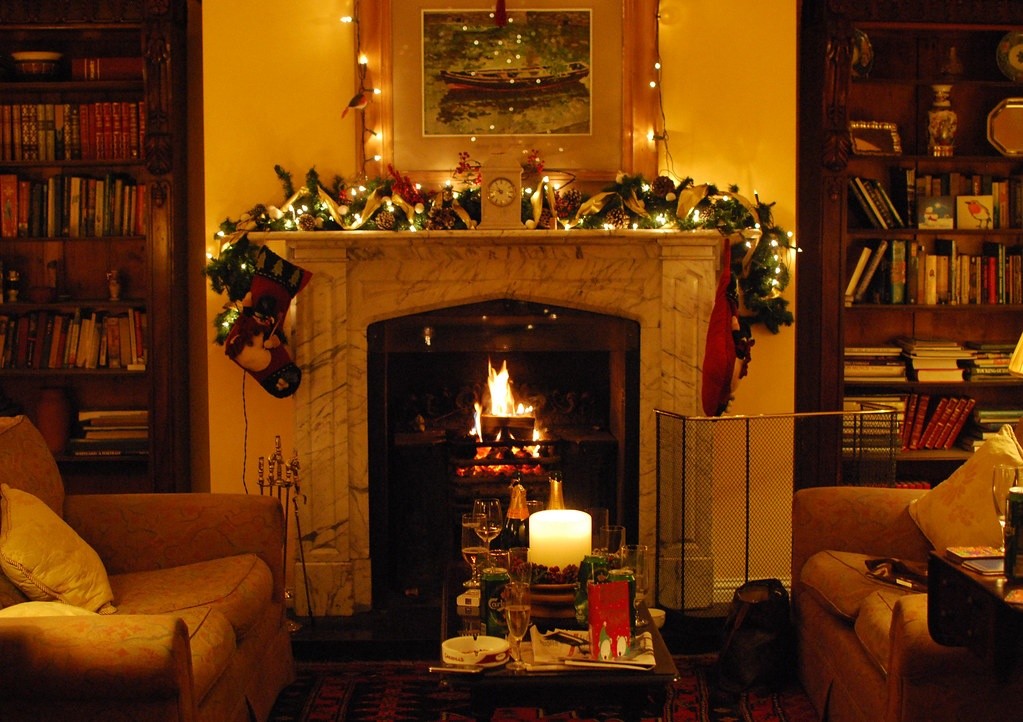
[381,0,633,181]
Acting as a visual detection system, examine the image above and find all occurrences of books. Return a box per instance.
[844,238,1023,304]
[71,57,143,81]
[848,479,931,491]
[0,309,148,370]
[66,410,151,457]
[0,101,147,163]
[844,337,1023,383]
[848,167,1023,229]
[843,393,1023,454]
[0,174,146,238]
[945,545,1005,575]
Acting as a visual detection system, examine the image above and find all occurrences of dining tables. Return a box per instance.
[440,560,678,722]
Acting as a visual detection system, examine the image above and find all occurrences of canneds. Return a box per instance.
[1004,487,1023,586]
[574,555,610,626]
[478,567,510,638]
[607,569,637,644]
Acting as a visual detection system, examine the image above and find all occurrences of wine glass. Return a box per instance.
[472,499,503,551]
[505,582,532,671]
[992,464,1023,552]
[621,545,648,628]
[461,514,488,588]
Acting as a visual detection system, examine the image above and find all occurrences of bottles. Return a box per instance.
[500,481,530,551]
[546,471,566,511]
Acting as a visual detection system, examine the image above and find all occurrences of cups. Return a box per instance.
[488,549,510,576]
[601,525,627,569]
[526,501,544,516]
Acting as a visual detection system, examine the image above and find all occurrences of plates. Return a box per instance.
[996,31,1023,82]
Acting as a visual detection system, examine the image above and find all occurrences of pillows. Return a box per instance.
[0,483,119,616]
[909,424,1023,554]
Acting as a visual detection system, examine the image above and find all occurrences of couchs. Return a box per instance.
[791,485,996,722]
[0,414,300,722]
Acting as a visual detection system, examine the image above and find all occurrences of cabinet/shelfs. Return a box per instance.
[0,0,210,493]
[797,2,1023,480]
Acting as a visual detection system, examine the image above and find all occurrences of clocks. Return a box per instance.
[488,178,518,205]
